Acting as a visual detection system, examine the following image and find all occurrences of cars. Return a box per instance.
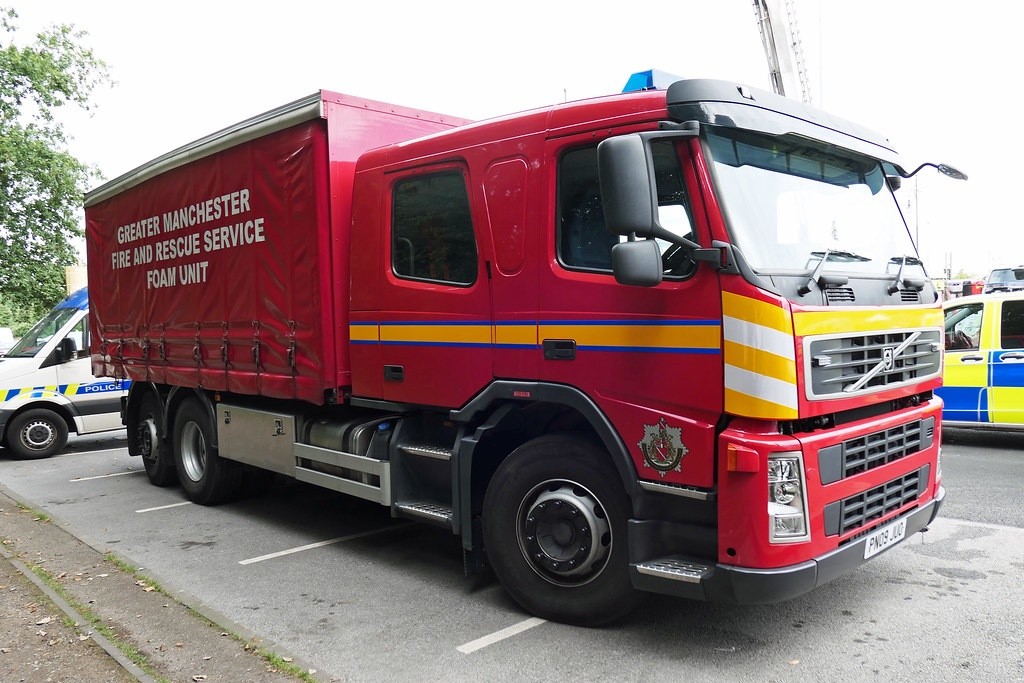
[933,292,1024,434]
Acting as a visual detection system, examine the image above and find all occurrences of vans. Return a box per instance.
[983,268,1024,294]
[0,282,133,461]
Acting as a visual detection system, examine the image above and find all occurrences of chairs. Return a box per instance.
[564,186,620,271]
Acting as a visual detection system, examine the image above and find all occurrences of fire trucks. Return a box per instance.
[85,68,970,627]
